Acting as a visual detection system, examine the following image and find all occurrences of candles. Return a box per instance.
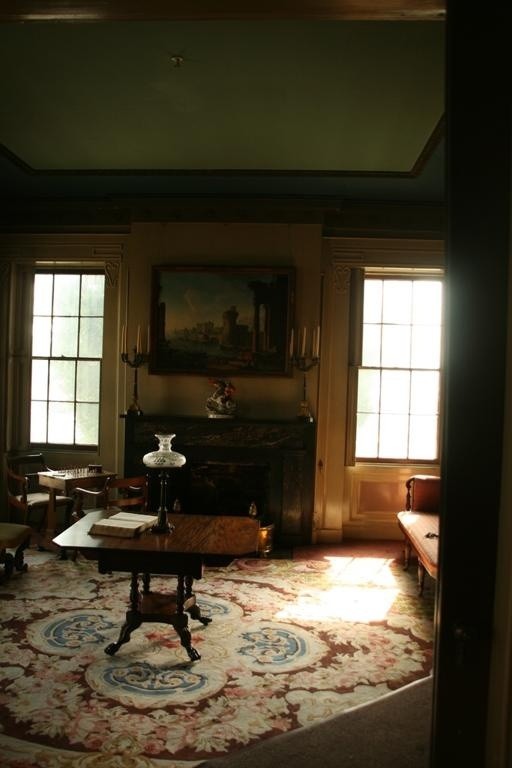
[121,326,150,354]
[289,326,321,357]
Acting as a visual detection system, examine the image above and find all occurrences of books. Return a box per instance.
[109,511,159,527]
[87,518,146,537]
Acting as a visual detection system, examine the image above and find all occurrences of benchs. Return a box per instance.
[398,475,439,596]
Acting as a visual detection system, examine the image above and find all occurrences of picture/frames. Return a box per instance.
[147,262,298,379]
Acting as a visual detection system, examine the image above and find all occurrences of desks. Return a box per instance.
[53,510,260,660]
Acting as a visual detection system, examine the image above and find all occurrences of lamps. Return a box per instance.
[142,434,186,535]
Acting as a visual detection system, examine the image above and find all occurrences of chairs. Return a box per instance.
[3,452,149,573]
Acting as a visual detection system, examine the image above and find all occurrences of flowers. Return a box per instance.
[208,380,238,400]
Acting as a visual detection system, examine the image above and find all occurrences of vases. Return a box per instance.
[205,393,240,420]
